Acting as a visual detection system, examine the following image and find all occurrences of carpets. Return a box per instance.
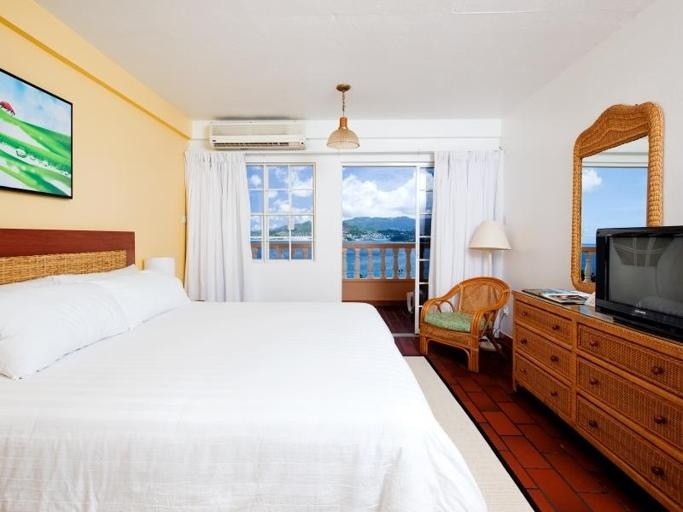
[402,354,541,511]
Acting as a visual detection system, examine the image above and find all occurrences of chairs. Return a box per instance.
[420,277,510,373]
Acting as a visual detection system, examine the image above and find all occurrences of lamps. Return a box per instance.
[470,223,511,352]
[144,256,175,275]
[327,85,359,147]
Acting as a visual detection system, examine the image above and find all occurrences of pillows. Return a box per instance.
[86,265,190,324]
[0,273,128,380]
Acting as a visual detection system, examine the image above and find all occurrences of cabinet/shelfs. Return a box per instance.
[573,310,683,512]
[510,289,573,421]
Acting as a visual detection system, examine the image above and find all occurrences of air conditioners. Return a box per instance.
[209,122,307,152]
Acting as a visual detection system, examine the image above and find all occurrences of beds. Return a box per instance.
[0,229,486,512]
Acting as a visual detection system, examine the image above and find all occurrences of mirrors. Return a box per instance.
[570,103,663,295]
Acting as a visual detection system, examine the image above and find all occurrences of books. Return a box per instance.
[522,288,587,303]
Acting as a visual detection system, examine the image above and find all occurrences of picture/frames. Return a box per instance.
[0,68,73,200]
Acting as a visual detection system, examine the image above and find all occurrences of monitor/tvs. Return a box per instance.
[595,225,683,343]
